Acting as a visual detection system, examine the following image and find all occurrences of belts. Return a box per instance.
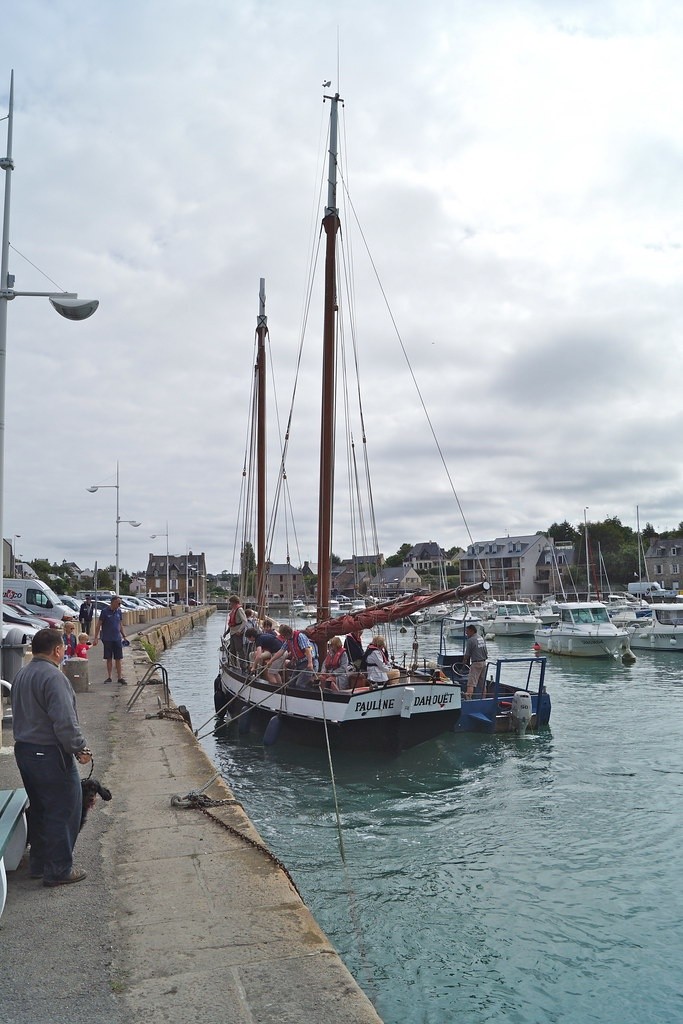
[472,660,484,662]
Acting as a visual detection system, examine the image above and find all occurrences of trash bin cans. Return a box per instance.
[0,628,29,697]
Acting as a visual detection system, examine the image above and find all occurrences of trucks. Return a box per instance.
[628,581,679,598]
[3,578,78,621]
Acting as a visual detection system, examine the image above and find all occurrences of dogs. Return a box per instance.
[24,780,112,846]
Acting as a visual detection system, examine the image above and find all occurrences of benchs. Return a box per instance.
[0,789,29,914]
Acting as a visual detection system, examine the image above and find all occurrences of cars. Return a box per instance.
[180,597,201,606]
[0,602,65,644]
[57,594,177,618]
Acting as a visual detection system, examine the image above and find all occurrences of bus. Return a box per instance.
[146,592,176,603]
[76,590,116,598]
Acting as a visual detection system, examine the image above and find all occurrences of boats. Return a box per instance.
[416,615,551,735]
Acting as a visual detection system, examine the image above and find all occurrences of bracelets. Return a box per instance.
[123,636,127,637]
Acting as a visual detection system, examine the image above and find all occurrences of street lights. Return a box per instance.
[86,459,142,595]
[149,520,169,607]
[0,69,100,577]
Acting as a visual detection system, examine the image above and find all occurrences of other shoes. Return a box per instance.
[104,678,112,684]
[42,868,87,886]
[31,871,43,878]
[118,678,127,684]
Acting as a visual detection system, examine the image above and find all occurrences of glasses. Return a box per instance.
[115,602,120,605]
[69,628,75,630]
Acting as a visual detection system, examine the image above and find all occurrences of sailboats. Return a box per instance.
[291,542,543,640]
[619,505,683,651]
[533,509,649,658]
[214,92,462,753]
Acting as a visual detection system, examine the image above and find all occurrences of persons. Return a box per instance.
[10,627,92,888]
[361,635,401,685]
[345,626,365,671]
[79,595,94,644]
[319,635,350,691]
[71,631,95,659]
[224,595,258,676]
[92,597,128,685]
[61,620,77,659]
[245,617,291,687]
[462,625,488,701]
[278,624,319,689]
